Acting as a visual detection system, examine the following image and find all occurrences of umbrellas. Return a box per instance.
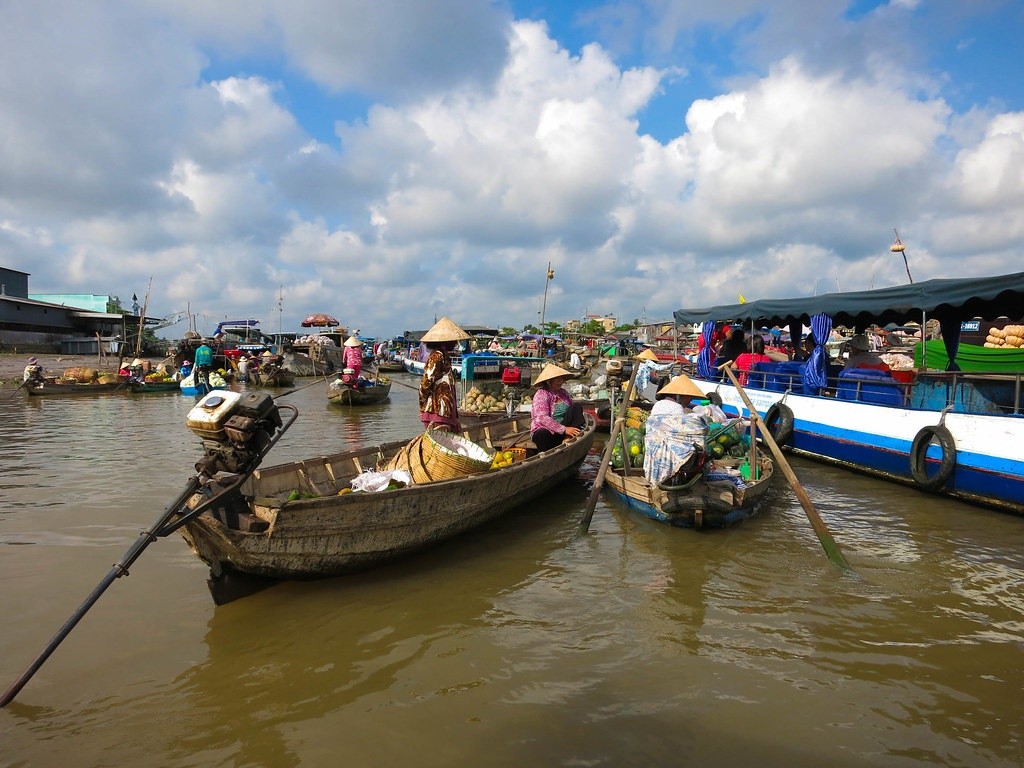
[301,312,340,333]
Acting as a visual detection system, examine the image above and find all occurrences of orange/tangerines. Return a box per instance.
[489,451,515,469]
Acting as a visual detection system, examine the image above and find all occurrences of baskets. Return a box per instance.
[376,448,394,472]
[502,441,527,462]
[385,421,496,483]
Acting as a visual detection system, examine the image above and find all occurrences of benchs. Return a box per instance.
[747,362,779,389]
[771,362,806,394]
[836,369,887,393]
[836,373,904,405]
[491,433,537,449]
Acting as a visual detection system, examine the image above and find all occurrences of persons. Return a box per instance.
[119,357,143,377]
[530,364,581,453]
[343,335,365,388]
[180,360,191,378]
[24,357,47,385]
[373,340,395,365]
[517,336,526,353]
[238,350,272,383]
[644,375,709,472]
[489,337,500,349]
[570,348,581,369]
[632,348,681,394]
[719,319,944,398]
[213,324,224,342]
[195,337,213,393]
[620,339,633,356]
[418,315,472,432]
[540,338,565,359]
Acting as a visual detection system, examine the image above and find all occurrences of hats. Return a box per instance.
[493,338,498,341]
[29,357,38,365]
[805,333,817,348]
[182,360,191,366]
[239,356,247,362]
[420,318,472,343]
[263,350,273,357]
[658,375,707,398]
[638,350,659,362]
[570,349,575,352]
[850,335,872,351]
[201,338,209,343]
[343,336,363,347]
[120,362,130,369]
[534,364,575,387]
[519,337,523,339]
[131,358,143,367]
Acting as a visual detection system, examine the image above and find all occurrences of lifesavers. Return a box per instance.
[761,403,794,448]
[655,376,670,399]
[910,426,956,490]
[411,363,414,370]
[706,392,722,408]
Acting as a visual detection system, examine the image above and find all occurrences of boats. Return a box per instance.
[666,271,1024,515]
[605,398,775,532]
[249,366,297,389]
[400,325,501,379]
[456,354,553,427]
[324,370,392,405]
[22,374,130,396]
[179,370,228,397]
[502,323,921,429]
[281,352,336,376]
[376,358,405,372]
[131,378,181,393]
[172,406,597,600]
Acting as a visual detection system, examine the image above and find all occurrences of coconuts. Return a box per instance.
[462,382,534,413]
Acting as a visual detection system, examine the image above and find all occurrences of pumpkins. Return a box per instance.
[983,325,1024,348]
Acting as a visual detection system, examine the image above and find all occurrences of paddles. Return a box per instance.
[345,361,418,390]
[717,359,854,566]
[579,356,647,533]
[272,367,343,400]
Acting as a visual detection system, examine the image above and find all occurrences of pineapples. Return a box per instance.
[610,403,646,430]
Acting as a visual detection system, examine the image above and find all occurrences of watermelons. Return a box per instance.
[608,423,647,468]
[704,430,749,461]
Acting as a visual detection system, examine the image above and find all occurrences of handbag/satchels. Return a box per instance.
[343,368,355,374]
